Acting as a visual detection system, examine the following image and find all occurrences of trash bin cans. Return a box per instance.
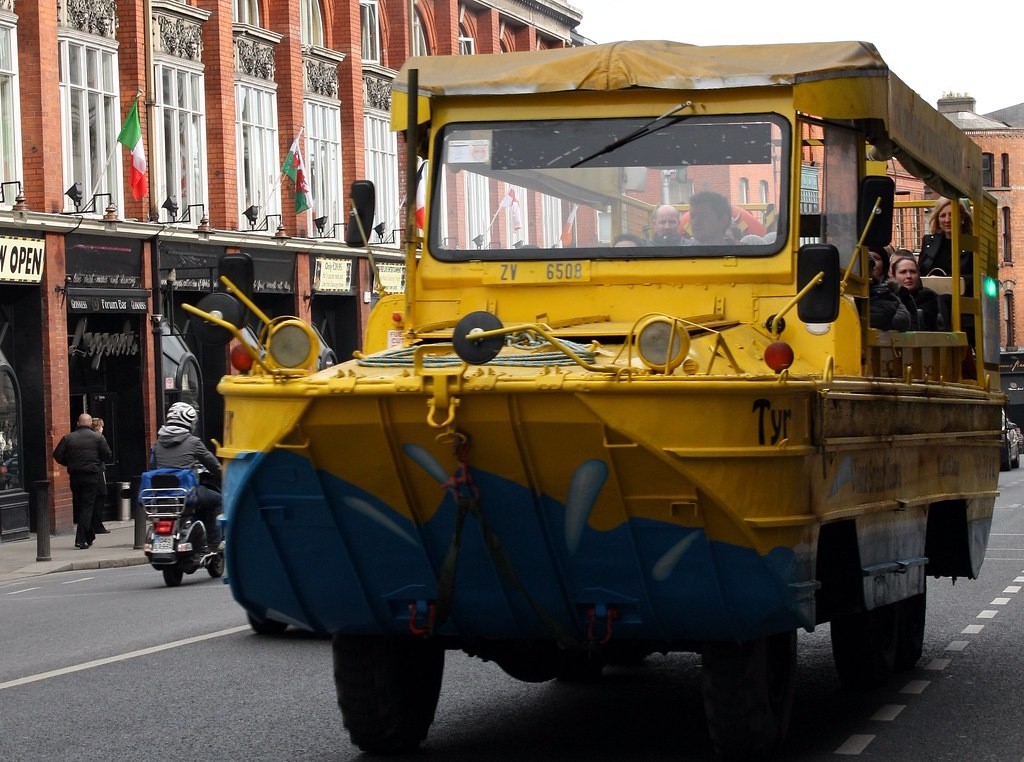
[116,482,132,521]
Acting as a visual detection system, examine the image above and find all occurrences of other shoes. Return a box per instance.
[95,528,110,533]
[206,540,227,551]
[75,540,90,549]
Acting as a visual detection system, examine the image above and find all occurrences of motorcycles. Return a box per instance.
[141,469,226,587]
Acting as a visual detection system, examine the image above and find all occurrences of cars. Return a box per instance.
[1001,409,1020,472]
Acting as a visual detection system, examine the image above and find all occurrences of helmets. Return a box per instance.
[166,402,198,434]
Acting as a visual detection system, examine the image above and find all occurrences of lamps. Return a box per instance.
[98,204,123,234]
[271,223,291,248]
[11,193,28,227]
[161,194,179,218]
[374,221,387,239]
[312,215,327,234]
[62,181,85,208]
[243,204,260,227]
[193,216,215,244]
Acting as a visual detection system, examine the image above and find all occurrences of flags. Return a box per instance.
[561,206,577,247]
[116,94,147,202]
[500,188,522,232]
[281,138,313,214]
[415,162,425,230]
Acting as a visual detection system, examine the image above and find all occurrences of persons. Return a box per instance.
[154,402,225,551]
[887,249,915,280]
[854,246,911,332]
[917,195,973,333]
[892,257,945,332]
[681,192,740,246]
[92,418,110,534]
[53,413,111,549]
[652,205,682,246]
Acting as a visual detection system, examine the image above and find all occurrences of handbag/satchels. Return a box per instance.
[138,468,200,504]
